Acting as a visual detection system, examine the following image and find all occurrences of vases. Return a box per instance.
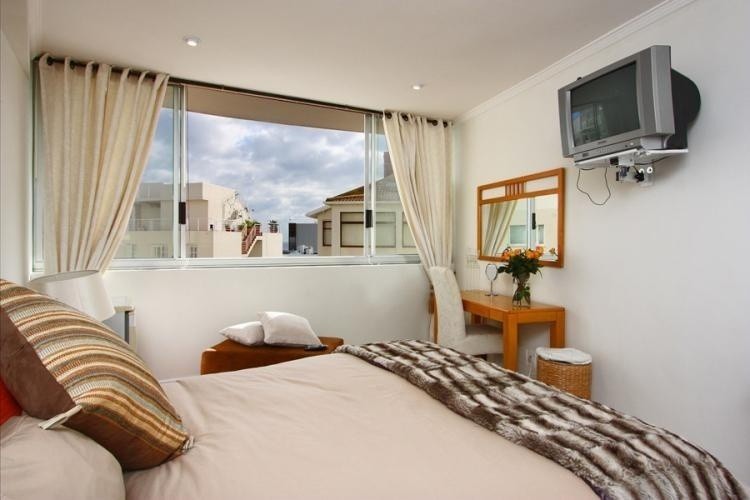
[512,284,531,308]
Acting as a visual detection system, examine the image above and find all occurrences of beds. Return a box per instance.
[0,277,750,500]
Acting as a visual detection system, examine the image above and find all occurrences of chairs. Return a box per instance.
[429,267,504,356]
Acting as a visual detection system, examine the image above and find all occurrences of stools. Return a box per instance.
[200,336,344,374]
[535,347,592,400]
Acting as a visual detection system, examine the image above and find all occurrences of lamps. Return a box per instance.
[26,270,115,324]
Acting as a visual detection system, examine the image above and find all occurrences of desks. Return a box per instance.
[433,288,566,372]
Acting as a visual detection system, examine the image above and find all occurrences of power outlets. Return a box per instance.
[528,350,536,368]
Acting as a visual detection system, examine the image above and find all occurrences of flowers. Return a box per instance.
[497,244,545,305]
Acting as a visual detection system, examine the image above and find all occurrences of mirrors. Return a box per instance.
[477,168,566,269]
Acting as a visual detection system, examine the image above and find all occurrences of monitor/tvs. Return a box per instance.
[557,43,702,162]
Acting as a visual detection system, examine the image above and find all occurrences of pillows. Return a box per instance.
[0,416,128,500]
[217,321,264,346]
[0,277,195,473]
[258,311,322,347]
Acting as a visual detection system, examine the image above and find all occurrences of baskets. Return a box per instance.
[537,358,592,400]
